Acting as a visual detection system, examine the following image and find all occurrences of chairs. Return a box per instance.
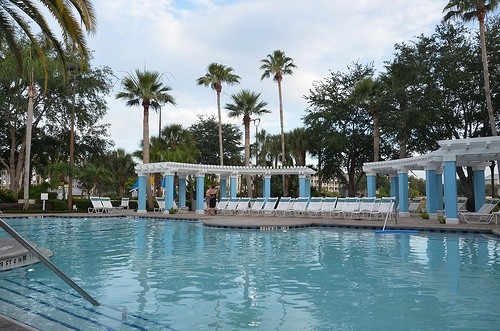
[88,197,124,214]
[205,195,395,220]
[154,197,180,212]
[436,196,469,220]
[118,197,130,210]
[408,198,424,217]
[460,198,500,224]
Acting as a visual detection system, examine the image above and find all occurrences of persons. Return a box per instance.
[209,185,218,216]
